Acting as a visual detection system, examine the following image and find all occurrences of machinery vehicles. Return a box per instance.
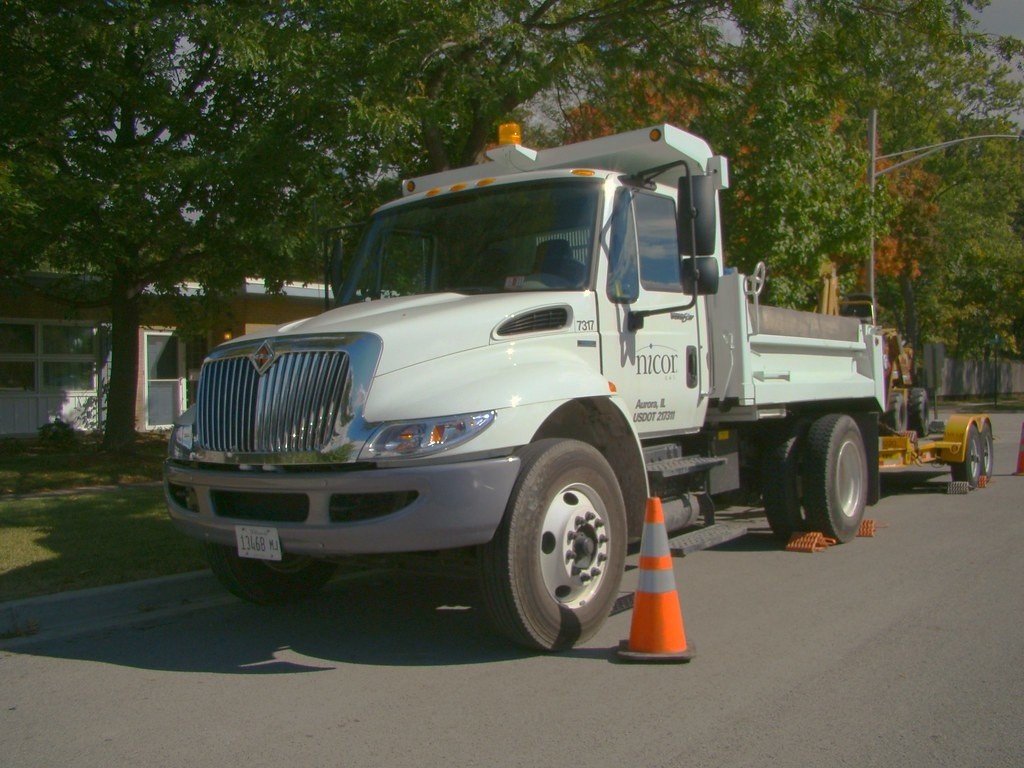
[803,248,996,491]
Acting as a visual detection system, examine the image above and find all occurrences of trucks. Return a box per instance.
[163,120,897,655]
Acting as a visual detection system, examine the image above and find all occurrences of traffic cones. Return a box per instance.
[1010,420,1024,474]
[616,494,696,662]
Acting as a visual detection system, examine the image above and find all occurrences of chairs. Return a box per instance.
[530,238,584,277]
[455,248,512,279]
